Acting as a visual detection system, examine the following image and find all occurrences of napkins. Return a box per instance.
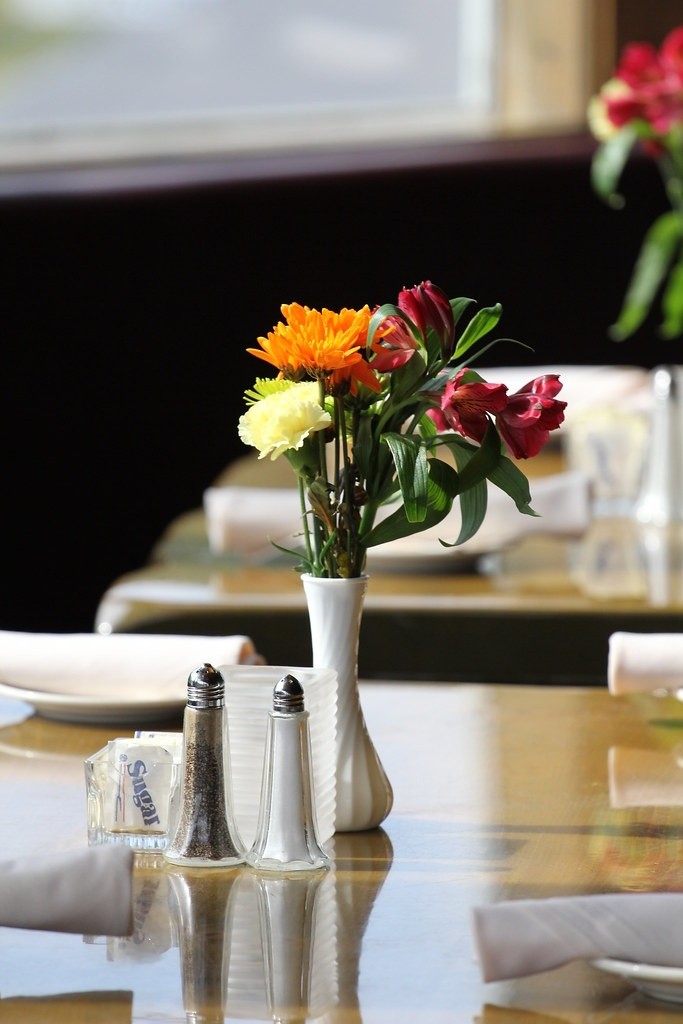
[202,473,593,557]
[608,632,683,694]
[0,630,266,697]
[471,893,683,984]
[608,745,683,809]
[0,846,136,937]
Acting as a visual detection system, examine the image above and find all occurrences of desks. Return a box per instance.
[0,365,683,1024]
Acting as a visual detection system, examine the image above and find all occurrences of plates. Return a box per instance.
[587,957,683,1004]
[365,543,486,574]
[0,681,189,724]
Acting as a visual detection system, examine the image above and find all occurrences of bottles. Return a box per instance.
[250,867,328,1024]
[245,673,331,872]
[165,865,242,1024]
[163,664,248,868]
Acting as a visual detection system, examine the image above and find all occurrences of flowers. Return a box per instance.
[236,278,566,578]
[588,27,683,340]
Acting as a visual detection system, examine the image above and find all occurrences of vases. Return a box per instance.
[300,573,394,831]
